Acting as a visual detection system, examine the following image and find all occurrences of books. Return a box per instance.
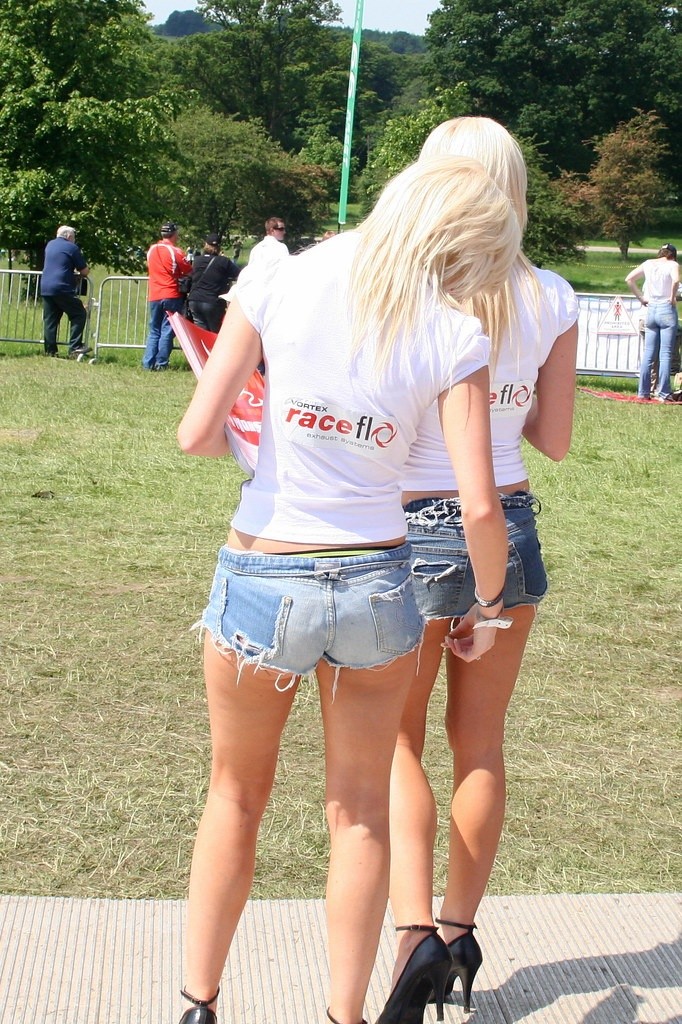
[164,310,265,478]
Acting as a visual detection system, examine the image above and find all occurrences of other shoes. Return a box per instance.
[659,393,678,404]
[70,344,93,354]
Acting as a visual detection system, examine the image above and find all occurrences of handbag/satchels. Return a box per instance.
[71,278,88,297]
[182,300,192,321]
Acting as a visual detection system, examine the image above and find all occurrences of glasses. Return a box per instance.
[273,227,285,232]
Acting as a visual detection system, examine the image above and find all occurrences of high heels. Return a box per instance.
[370,918,483,1024]
[177,992,221,1024]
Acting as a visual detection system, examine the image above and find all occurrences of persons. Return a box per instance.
[175,164,520,1023]
[41,226,93,357]
[625,243,679,401]
[374,116,581,1024]
[323,231,335,241]
[141,223,243,372]
[248,217,289,262]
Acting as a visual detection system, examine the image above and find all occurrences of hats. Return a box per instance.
[659,243,678,262]
[206,233,222,247]
[160,221,179,232]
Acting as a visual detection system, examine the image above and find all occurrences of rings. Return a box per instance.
[476,656,481,661]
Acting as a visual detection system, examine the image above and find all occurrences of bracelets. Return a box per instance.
[474,587,505,608]
[472,604,514,631]
